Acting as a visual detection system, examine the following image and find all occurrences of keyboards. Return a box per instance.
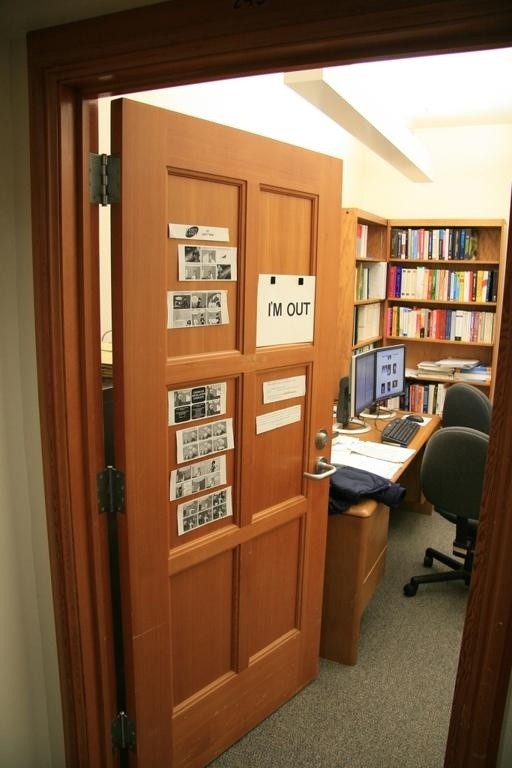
[380,418,421,446]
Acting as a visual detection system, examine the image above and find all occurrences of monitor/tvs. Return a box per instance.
[359,345,407,420]
[330,350,376,435]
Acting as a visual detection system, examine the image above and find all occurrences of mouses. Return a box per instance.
[406,413,424,422]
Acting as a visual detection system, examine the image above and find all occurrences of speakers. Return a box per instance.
[336,377,349,428]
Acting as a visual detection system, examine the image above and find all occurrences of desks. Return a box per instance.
[320,410,442,670]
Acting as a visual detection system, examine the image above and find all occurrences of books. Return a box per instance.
[346,223,499,424]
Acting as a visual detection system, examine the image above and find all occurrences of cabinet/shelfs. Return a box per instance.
[384,218,508,427]
[335,212,388,407]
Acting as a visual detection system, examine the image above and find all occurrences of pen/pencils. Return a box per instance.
[383,441,407,448]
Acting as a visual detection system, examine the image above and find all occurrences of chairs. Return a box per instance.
[441,383,491,434]
[403,426,489,596]
